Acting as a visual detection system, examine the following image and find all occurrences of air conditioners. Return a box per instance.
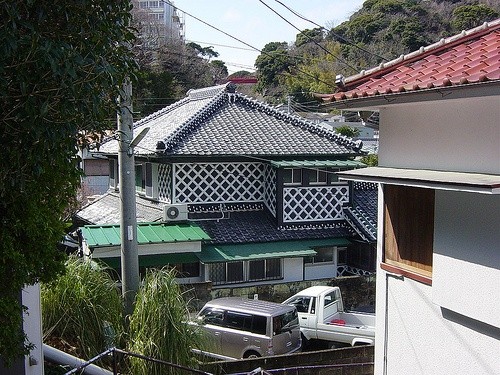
[162,203,188,222]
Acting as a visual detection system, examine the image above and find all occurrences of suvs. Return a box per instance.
[179,296,301,360]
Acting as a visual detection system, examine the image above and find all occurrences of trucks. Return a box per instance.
[281,286,376,348]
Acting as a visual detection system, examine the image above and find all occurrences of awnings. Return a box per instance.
[195,240,317,263]
